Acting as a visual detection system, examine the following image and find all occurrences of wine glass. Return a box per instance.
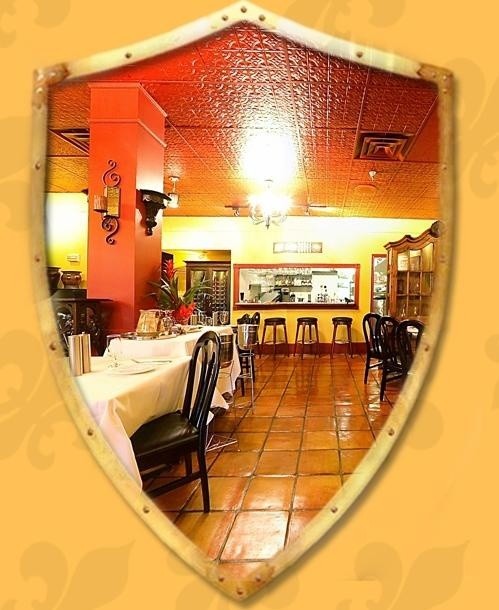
[160,308,176,335]
[105,333,123,370]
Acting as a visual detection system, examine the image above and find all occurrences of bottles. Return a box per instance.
[318,285,328,302]
[67,331,93,376]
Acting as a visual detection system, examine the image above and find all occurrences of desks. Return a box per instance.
[62,323,233,492]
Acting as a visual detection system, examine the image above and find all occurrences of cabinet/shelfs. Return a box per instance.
[383,228,438,326]
[183,260,231,318]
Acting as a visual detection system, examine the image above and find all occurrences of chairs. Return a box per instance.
[362,313,425,401]
[129,330,223,513]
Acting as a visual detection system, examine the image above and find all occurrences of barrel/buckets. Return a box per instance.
[218,334,234,368]
[236,323,259,350]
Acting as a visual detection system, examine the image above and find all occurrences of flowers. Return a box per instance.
[142,258,215,311]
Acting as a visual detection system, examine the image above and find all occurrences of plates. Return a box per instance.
[107,364,156,375]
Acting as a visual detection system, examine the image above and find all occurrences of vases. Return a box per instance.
[47,266,83,289]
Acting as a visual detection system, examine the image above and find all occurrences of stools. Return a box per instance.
[259,317,353,360]
